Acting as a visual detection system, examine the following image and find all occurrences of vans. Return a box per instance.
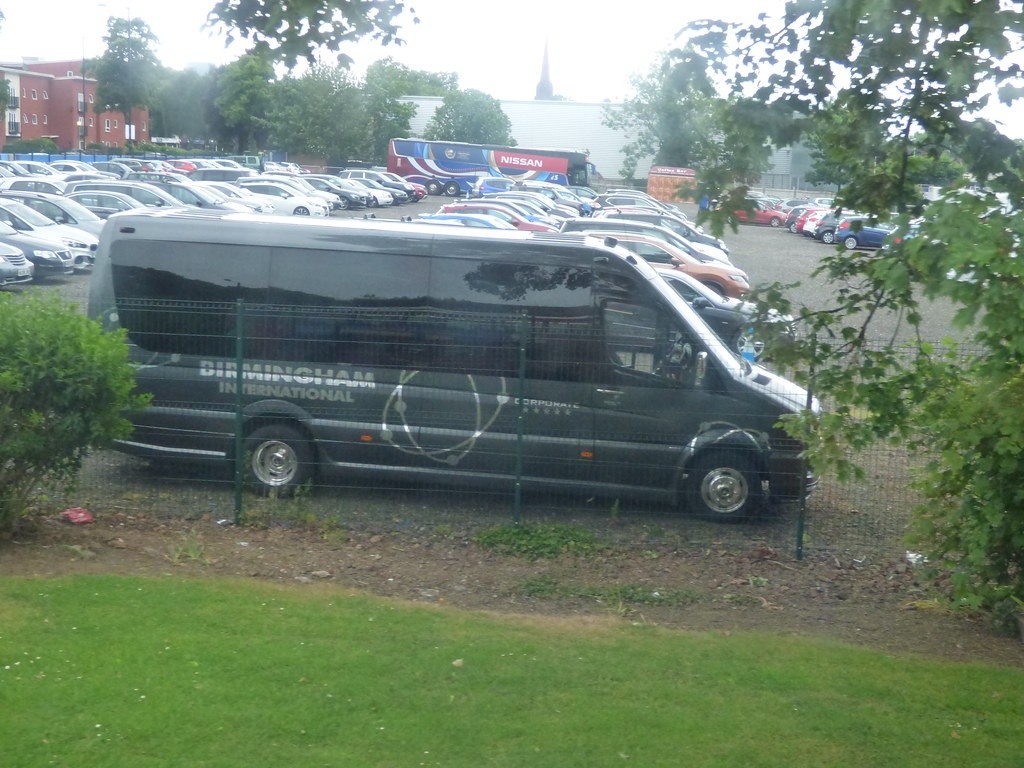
[85,203,824,525]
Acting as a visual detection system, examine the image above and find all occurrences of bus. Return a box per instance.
[386,137,596,198]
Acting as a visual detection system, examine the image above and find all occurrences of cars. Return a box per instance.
[394,182,950,366]
[0,153,429,216]
[62,190,150,219]
[0,197,100,273]
[0,220,76,280]
[0,242,35,291]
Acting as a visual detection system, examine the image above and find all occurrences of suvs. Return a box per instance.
[0,188,107,241]
[64,179,198,210]
